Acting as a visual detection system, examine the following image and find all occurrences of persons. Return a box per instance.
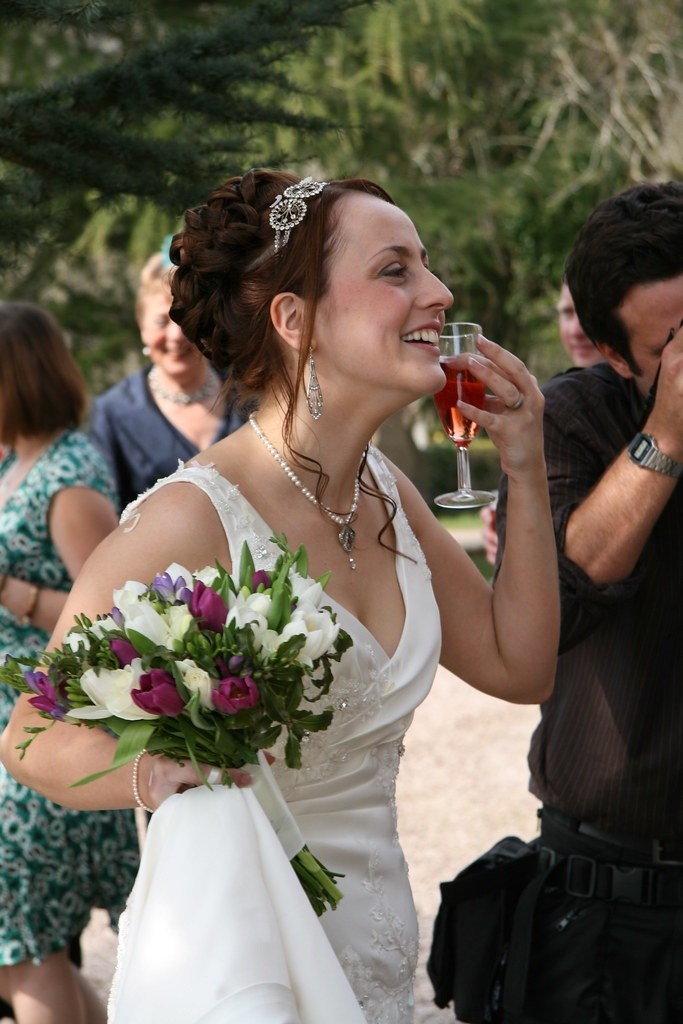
[481,182,682,1024]
[0,305,141,1024]
[91,254,263,520]
[0,171,560,1023]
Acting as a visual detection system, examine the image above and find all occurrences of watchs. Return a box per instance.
[629,433,682,480]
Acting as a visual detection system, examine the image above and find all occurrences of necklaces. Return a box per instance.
[250,409,362,569]
[147,363,217,404]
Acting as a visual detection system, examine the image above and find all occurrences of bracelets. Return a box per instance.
[131,748,155,816]
[0,573,9,594]
[19,584,42,631]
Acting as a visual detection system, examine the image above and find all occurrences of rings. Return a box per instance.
[505,392,524,409]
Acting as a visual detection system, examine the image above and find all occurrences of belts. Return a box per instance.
[542,800,683,868]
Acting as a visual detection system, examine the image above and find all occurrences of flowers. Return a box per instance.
[0,532,345,913]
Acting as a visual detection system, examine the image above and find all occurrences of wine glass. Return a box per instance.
[430,321,495,509]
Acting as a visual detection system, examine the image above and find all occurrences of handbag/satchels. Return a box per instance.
[427,837,546,1024]
